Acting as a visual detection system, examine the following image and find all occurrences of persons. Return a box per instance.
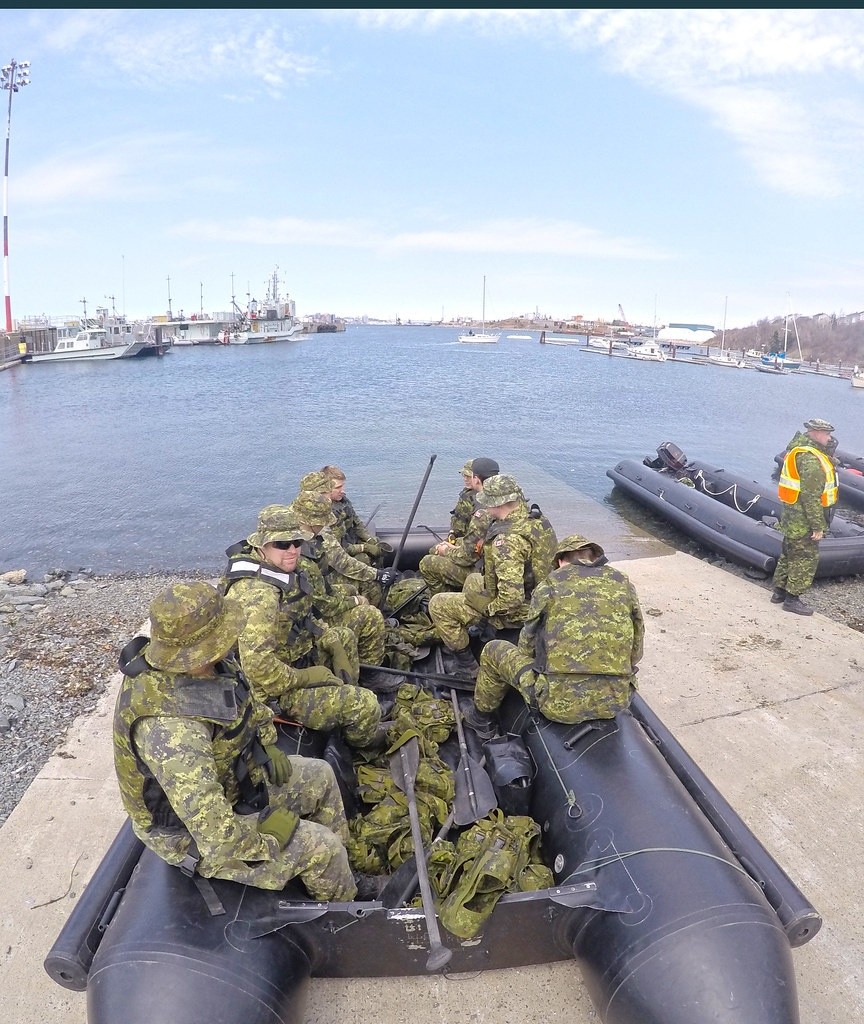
[216,503,395,748]
[770,418,839,616]
[299,465,395,608]
[289,491,387,667]
[419,457,500,596]
[458,533,645,739]
[428,474,559,678]
[112,581,389,902]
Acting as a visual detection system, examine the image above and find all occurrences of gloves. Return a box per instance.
[464,590,496,615]
[257,804,300,853]
[324,641,357,687]
[350,582,370,606]
[262,745,292,787]
[363,539,379,559]
[376,566,402,584]
[295,666,344,688]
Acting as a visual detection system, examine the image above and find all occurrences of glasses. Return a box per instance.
[270,540,303,550]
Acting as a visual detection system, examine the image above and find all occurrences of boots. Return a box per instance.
[459,699,496,739]
[427,645,479,688]
[348,858,393,901]
[782,591,812,615]
[770,587,786,602]
[357,665,408,694]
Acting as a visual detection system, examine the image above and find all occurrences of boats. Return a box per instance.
[605,441,864,581]
[596,295,805,375]
[777,440,864,505]
[17,265,306,364]
[540,330,581,347]
[506,334,533,341]
[850,365,864,388]
[35,523,819,1011]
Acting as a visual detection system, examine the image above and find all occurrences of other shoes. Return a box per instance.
[384,617,400,630]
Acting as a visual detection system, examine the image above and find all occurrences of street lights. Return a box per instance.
[0,56,33,332]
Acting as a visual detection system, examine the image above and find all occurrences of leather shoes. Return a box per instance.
[366,700,399,750]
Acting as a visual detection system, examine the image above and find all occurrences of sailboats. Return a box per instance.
[458,275,505,344]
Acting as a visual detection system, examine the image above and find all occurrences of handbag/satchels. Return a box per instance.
[481,733,537,818]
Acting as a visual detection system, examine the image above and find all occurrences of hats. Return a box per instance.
[301,472,335,493]
[144,582,247,673]
[459,458,477,477]
[803,419,835,431]
[471,458,499,481]
[550,534,603,569]
[288,492,337,526]
[475,475,522,508]
[247,504,314,548]
[367,541,394,567]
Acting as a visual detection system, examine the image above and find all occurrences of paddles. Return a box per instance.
[358,663,476,692]
[376,733,501,908]
[450,688,498,826]
[365,501,386,529]
[389,736,452,971]
[377,454,437,610]
